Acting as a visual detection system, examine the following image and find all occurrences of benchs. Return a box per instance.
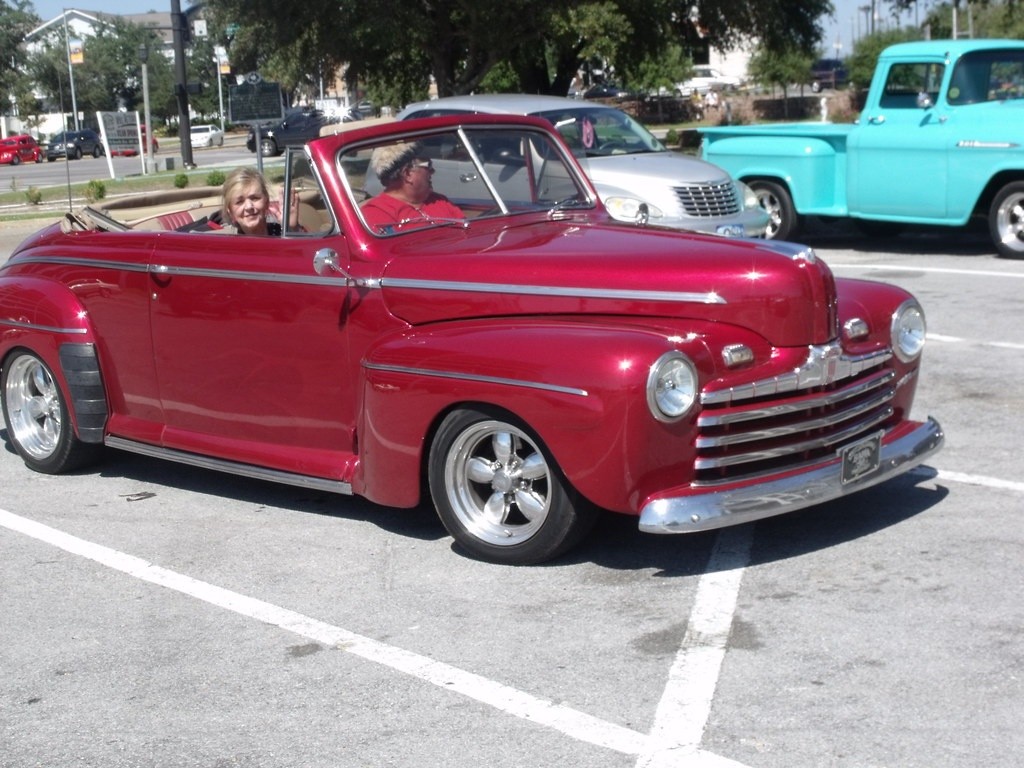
[125,198,325,234]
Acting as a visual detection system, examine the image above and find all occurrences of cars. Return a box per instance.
[582,84,627,99]
[362,93,772,242]
[246,108,365,158]
[0,133,45,166]
[46,128,102,162]
[645,64,745,102]
[98,122,159,157]
[804,58,853,94]
[189,123,225,148]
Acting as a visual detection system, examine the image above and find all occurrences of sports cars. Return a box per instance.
[0,112,945,567]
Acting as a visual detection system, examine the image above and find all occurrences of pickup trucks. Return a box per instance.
[695,38,1024,259]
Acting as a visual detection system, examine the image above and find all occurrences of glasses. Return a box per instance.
[412,161,432,170]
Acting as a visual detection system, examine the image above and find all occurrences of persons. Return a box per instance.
[690,88,718,121]
[356,140,466,236]
[813,79,821,93]
[213,167,308,236]
